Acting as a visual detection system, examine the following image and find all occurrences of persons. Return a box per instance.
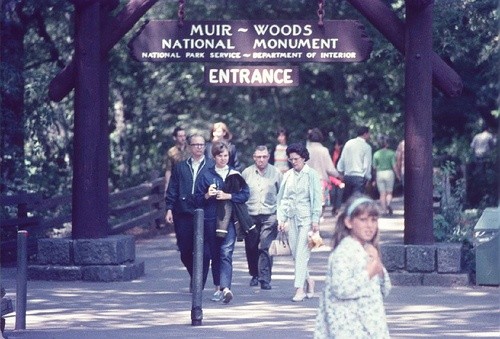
[313,193,393,339]
[470,122,498,160]
[163,121,406,305]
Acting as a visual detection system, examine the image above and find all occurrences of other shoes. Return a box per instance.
[250,276,258,286]
[261,282,271,289]
[293,293,306,301]
[384,213,388,216]
[306,279,314,298]
[212,290,223,301]
[387,205,393,214]
[222,290,233,303]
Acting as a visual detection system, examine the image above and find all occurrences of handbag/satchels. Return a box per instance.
[307,230,323,249]
[268,229,292,257]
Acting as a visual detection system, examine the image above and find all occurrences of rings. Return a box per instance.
[217,196,220,198]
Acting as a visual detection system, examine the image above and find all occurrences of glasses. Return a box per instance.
[190,144,206,147]
[288,158,299,162]
